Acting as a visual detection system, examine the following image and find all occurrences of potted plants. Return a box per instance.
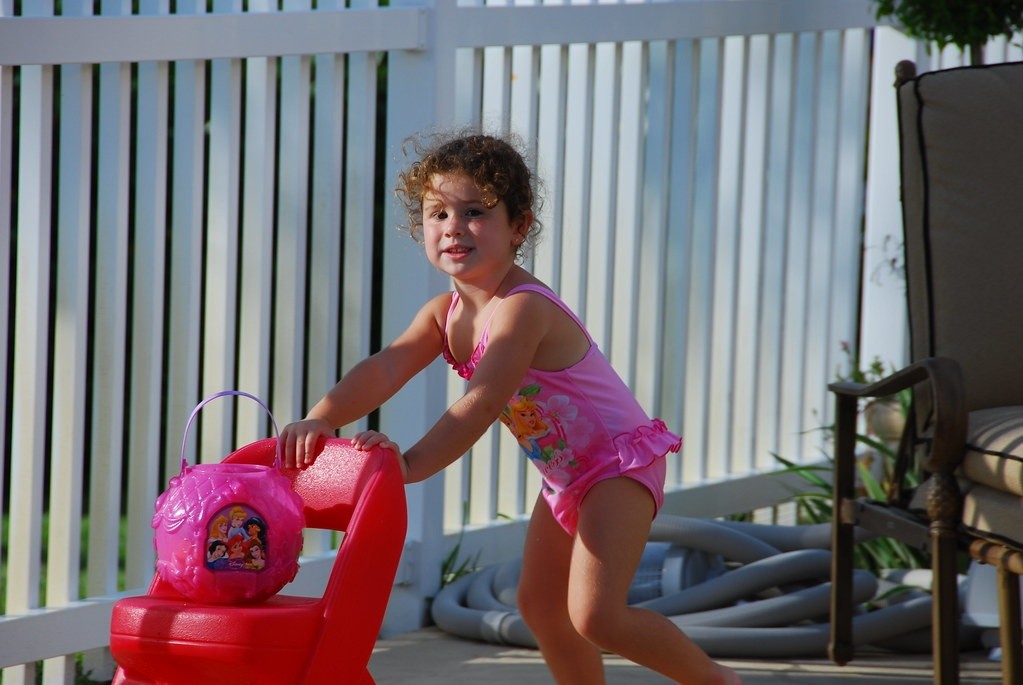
[865,0,1023,498]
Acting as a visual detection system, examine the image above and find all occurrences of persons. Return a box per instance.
[278,135,741,685]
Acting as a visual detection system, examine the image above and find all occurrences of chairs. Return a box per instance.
[826,59,1023,685]
[109,437,408,685]
